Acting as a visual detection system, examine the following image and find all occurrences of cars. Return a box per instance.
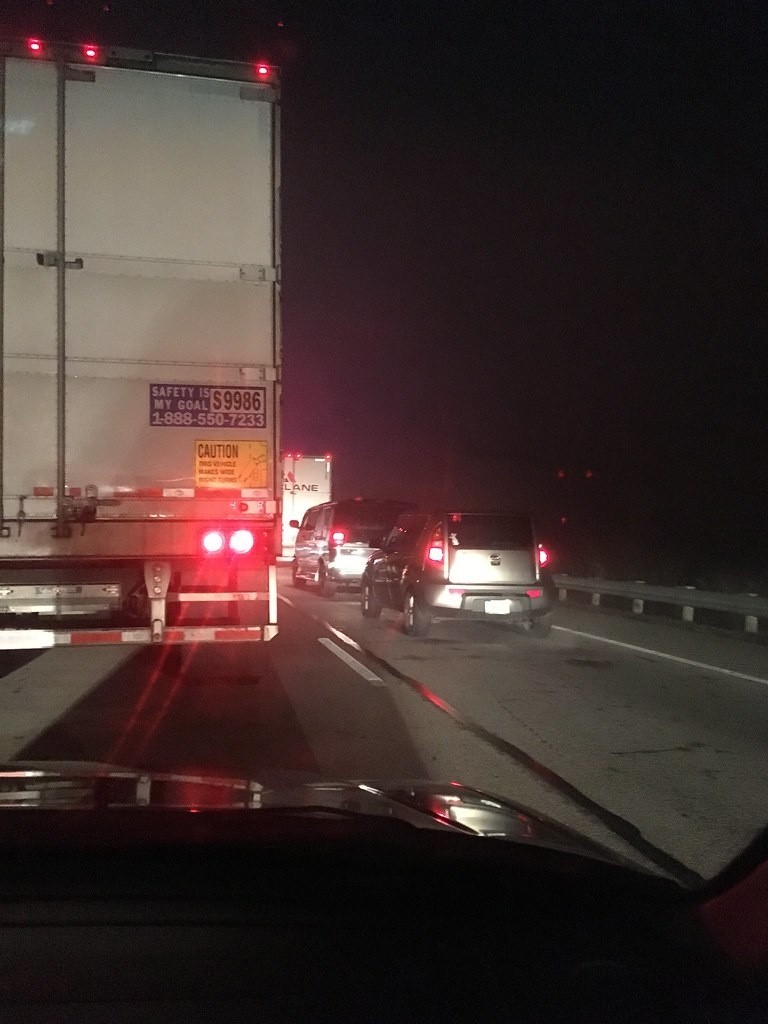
[360,509,557,636]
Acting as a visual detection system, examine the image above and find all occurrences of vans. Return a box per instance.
[287,497,414,596]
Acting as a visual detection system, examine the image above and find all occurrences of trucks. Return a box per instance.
[0,35,281,642]
[279,451,332,560]
